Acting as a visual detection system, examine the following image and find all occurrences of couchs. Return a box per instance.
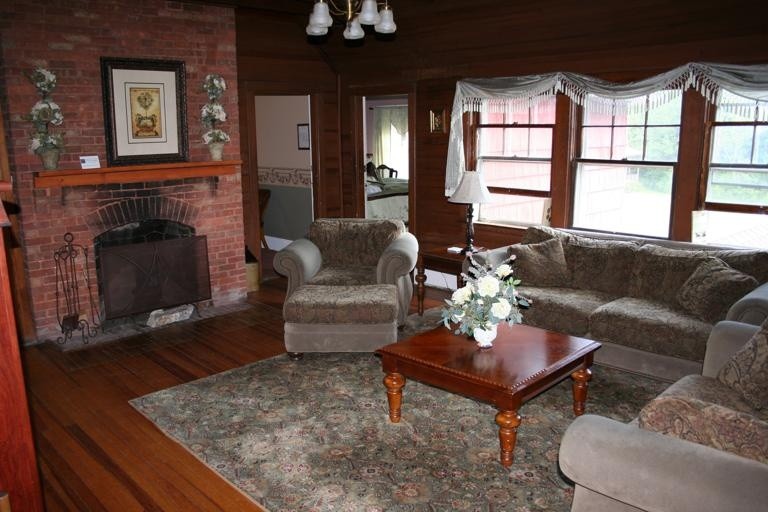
[462,222,768,364]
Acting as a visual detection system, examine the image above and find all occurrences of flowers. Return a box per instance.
[437,251,533,336]
[198,73,233,144]
[22,65,67,156]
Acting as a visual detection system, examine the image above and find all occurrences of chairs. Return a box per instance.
[272,215,419,361]
[558,314,768,511]
[375,163,399,178]
[258,186,272,249]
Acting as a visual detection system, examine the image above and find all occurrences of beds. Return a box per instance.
[366,178,410,229]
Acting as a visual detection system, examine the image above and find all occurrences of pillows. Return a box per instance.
[506,238,574,288]
[715,317,768,410]
[678,249,762,324]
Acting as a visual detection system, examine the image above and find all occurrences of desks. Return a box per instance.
[416,241,482,318]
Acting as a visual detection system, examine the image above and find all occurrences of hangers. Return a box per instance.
[366,154,374,164]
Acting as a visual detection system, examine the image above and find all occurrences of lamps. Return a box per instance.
[449,170,494,254]
[305,0,400,38]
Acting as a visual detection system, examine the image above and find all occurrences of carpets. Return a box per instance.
[129,317,675,512]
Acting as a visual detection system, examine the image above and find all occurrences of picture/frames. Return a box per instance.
[98,55,194,167]
[296,123,310,150]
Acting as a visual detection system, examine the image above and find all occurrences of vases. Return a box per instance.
[471,321,498,350]
[209,140,225,162]
[39,146,59,169]
